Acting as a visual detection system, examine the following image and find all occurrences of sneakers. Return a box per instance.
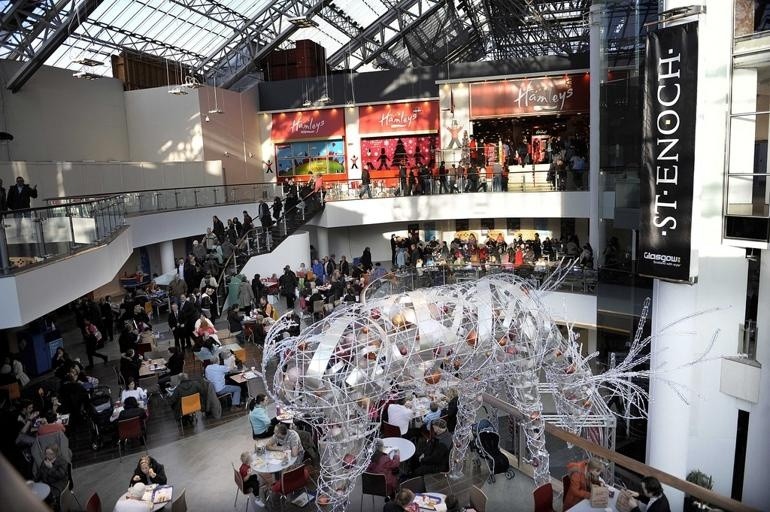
[102,355,108,367]
[85,362,95,370]
[230,407,243,413]
[254,499,265,508]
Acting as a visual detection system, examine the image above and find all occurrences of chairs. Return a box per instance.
[0,240,646,512]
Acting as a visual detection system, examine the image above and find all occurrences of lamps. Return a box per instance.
[67,0,333,114]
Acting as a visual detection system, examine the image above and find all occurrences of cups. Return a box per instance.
[608,487,615,498]
[148,358,152,363]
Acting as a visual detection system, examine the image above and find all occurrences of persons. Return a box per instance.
[239,450,266,509]
[33,445,68,512]
[364,436,401,496]
[391,231,593,289]
[194,334,242,409]
[444,493,477,511]
[547,132,590,193]
[560,458,615,511]
[446,119,465,150]
[358,165,373,198]
[470,135,555,168]
[2,346,90,460]
[394,156,510,196]
[278,245,388,326]
[75,280,202,447]
[128,452,167,487]
[624,475,671,512]
[258,171,328,248]
[222,272,300,374]
[398,417,453,482]
[383,484,417,511]
[1,175,38,238]
[167,209,256,352]
[253,424,306,484]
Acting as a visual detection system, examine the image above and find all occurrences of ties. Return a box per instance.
[175,313,178,321]
